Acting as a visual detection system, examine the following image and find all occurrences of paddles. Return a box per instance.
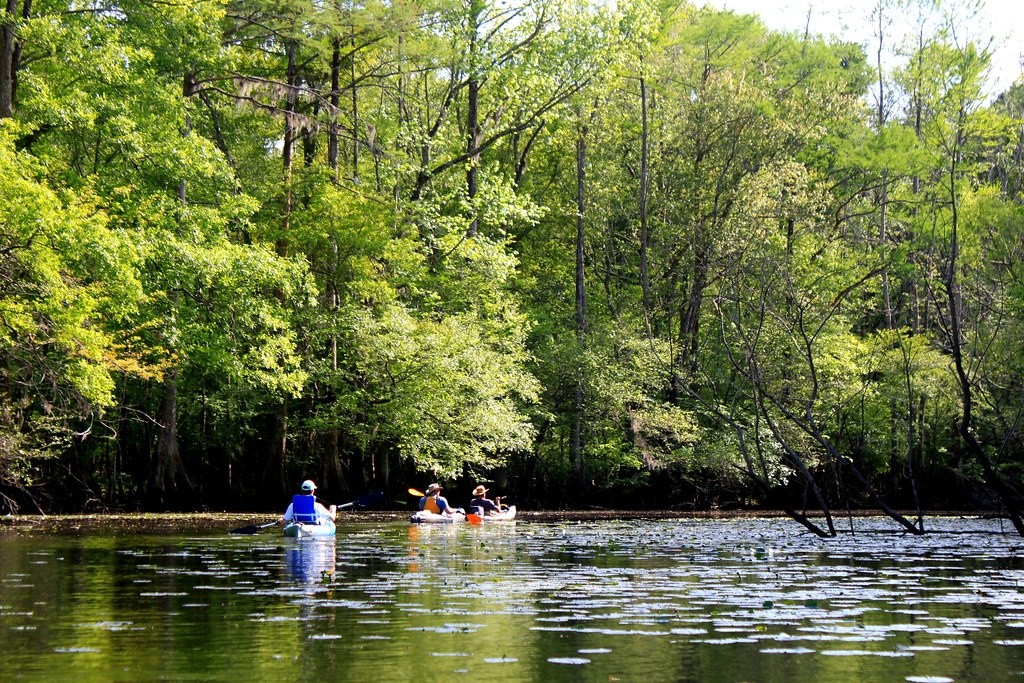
[225,489,379,535]
[408,488,482,523]
[491,495,508,503]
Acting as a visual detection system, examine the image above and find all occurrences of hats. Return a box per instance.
[426,483,443,494]
[473,485,489,496]
[302,480,317,490]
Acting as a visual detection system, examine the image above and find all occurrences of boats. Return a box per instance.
[410,508,466,523]
[466,506,516,524]
[282,516,336,538]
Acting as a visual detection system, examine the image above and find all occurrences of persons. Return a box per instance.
[418,484,462,516]
[278,478,338,526]
[470,485,502,518]
